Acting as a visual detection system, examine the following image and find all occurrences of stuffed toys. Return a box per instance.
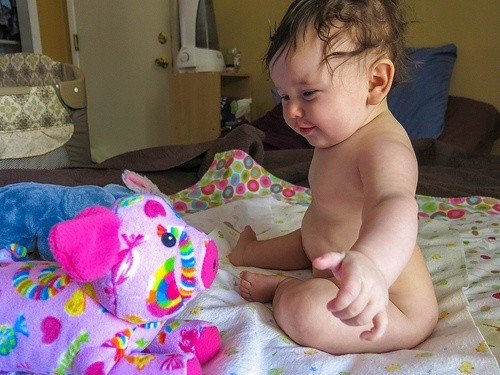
[0,165,222,375]
[0,180,188,263]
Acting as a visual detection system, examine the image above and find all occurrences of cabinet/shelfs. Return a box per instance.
[172,68,256,142]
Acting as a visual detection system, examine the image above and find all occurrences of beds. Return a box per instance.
[0,92,498,374]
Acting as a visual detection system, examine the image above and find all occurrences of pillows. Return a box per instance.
[388,42,457,142]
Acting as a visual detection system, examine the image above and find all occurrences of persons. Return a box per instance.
[227,1,440,355]
